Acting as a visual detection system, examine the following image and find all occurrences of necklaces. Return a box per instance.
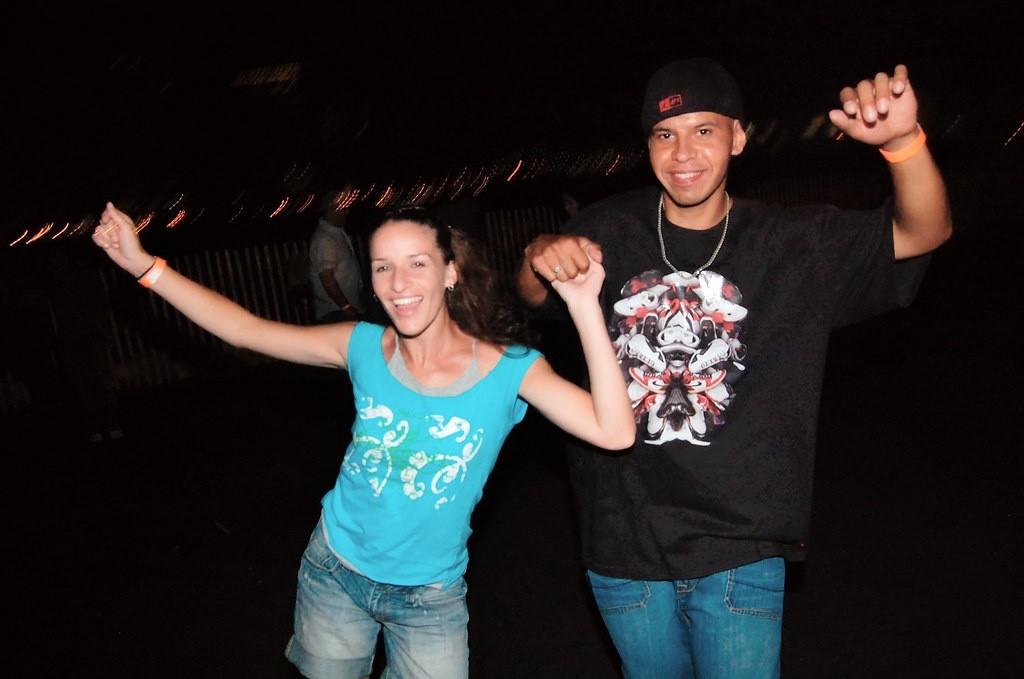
[657,191,730,279]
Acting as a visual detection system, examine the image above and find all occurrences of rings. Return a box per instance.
[554,266,561,273]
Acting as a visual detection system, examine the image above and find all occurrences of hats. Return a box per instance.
[641,56,746,139]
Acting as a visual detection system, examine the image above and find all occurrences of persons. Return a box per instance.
[310,192,364,321]
[516,57,952,678]
[92,202,636,679]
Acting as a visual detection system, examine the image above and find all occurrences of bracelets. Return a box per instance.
[138,256,166,287]
[343,304,351,310]
[879,124,925,163]
